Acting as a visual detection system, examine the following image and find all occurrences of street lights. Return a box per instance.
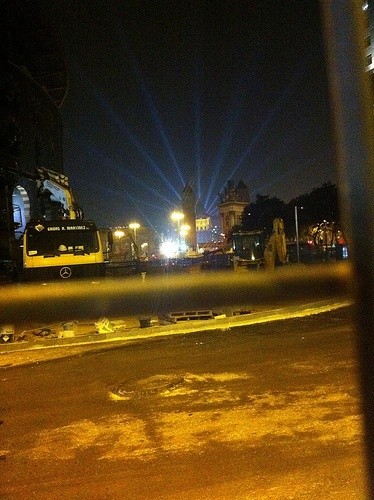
[128,223,140,244]
[172,213,184,261]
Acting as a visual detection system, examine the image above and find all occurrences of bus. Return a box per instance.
[13,219,113,283]
